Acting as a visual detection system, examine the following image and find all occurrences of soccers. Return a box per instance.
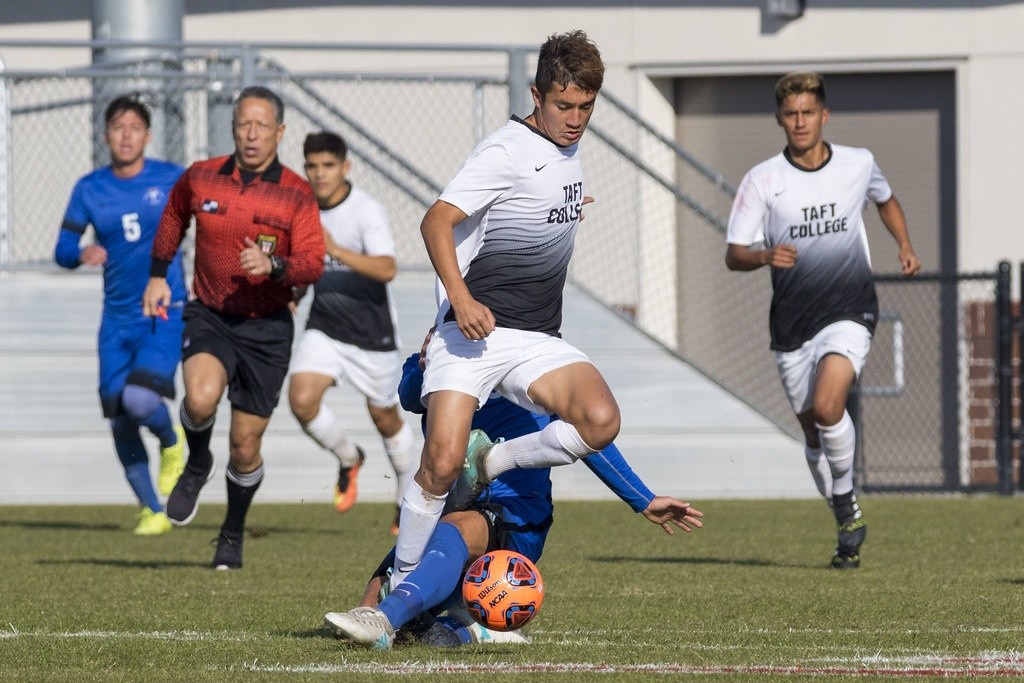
[461,549,545,632]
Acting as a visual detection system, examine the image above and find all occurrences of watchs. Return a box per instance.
[271,254,284,278]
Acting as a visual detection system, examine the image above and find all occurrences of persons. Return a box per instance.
[143,86,326,569]
[286,132,419,536]
[55,95,187,536]
[325,325,704,646]
[725,72,920,568]
[380,29,622,648]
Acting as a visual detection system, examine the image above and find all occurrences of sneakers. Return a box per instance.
[167,448,218,526]
[212,527,243,571]
[443,430,493,517]
[400,614,461,648]
[453,622,528,645]
[157,424,185,495]
[832,492,867,569]
[324,606,397,652]
[133,505,172,537]
[334,444,365,513]
